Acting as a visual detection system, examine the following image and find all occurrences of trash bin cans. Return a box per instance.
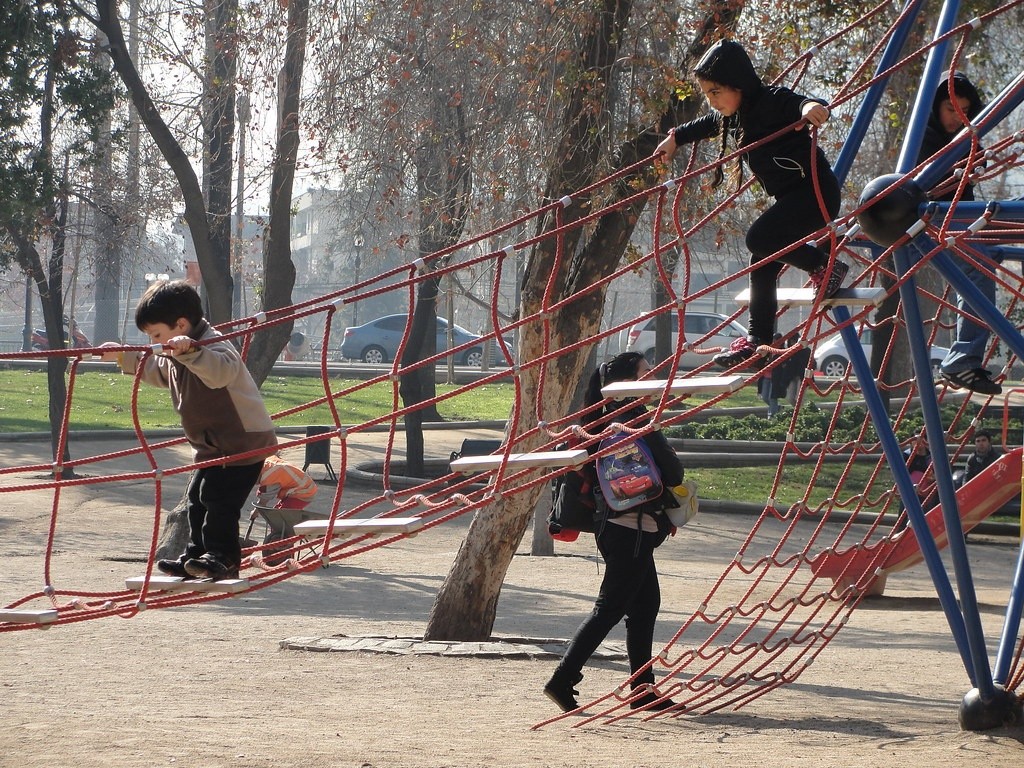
[301,425,339,484]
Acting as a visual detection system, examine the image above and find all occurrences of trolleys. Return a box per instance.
[253,502,347,567]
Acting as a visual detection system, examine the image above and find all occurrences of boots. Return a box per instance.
[630,673,686,710]
[543,666,582,714]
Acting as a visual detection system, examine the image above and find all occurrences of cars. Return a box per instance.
[813,323,950,377]
[625,311,749,371]
[341,313,514,367]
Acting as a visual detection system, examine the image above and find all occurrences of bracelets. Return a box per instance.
[667,128,676,137]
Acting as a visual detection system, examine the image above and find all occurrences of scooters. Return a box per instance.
[30,315,94,351]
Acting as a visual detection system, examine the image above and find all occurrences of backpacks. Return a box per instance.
[546,442,595,542]
[596,432,663,512]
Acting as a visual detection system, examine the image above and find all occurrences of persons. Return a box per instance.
[757,333,817,420]
[962,431,1001,543]
[653,39,849,369]
[544,352,687,714]
[99,280,278,580]
[249,454,317,521]
[911,69,1002,396]
[895,429,940,535]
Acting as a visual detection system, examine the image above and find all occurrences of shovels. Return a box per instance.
[238,516,259,559]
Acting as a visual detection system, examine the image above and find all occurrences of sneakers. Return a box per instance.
[158,560,189,577]
[712,335,767,371]
[942,367,1002,395]
[811,253,849,304]
[184,551,239,579]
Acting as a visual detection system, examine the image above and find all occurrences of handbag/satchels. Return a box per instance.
[911,471,929,498]
[663,480,698,527]
[654,511,675,548]
